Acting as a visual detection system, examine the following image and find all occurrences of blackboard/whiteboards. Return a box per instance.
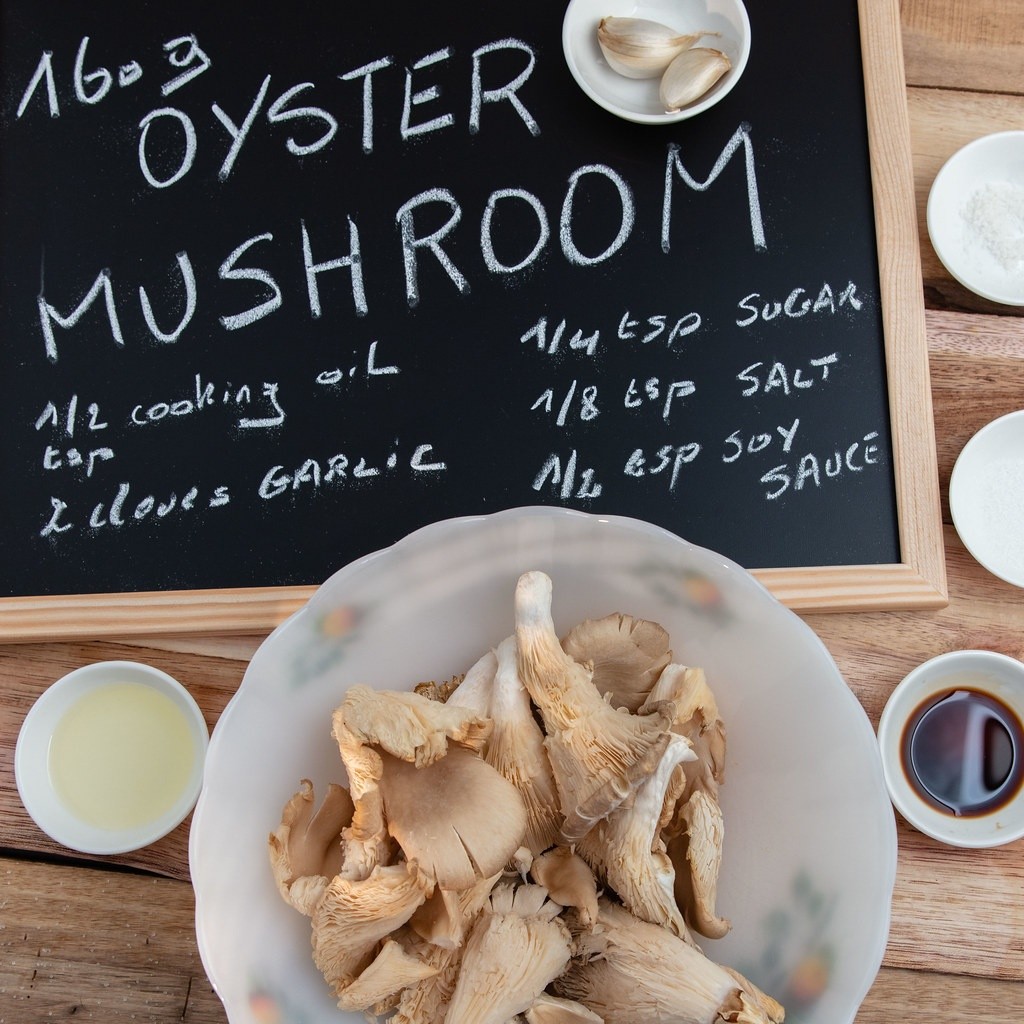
[1,0,952,648]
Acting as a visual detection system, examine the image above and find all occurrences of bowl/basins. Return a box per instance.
[189,505,898,1022]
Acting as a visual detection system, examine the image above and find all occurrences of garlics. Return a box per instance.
[599,15,732,106]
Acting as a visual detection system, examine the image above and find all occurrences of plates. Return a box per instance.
[13,659,209,856]
[562,1,751,124]
[877,648,1024,849]
[927,131,1023,306]
[948,409,1023,588]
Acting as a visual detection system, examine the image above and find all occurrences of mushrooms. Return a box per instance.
[271,570,788,1024]
